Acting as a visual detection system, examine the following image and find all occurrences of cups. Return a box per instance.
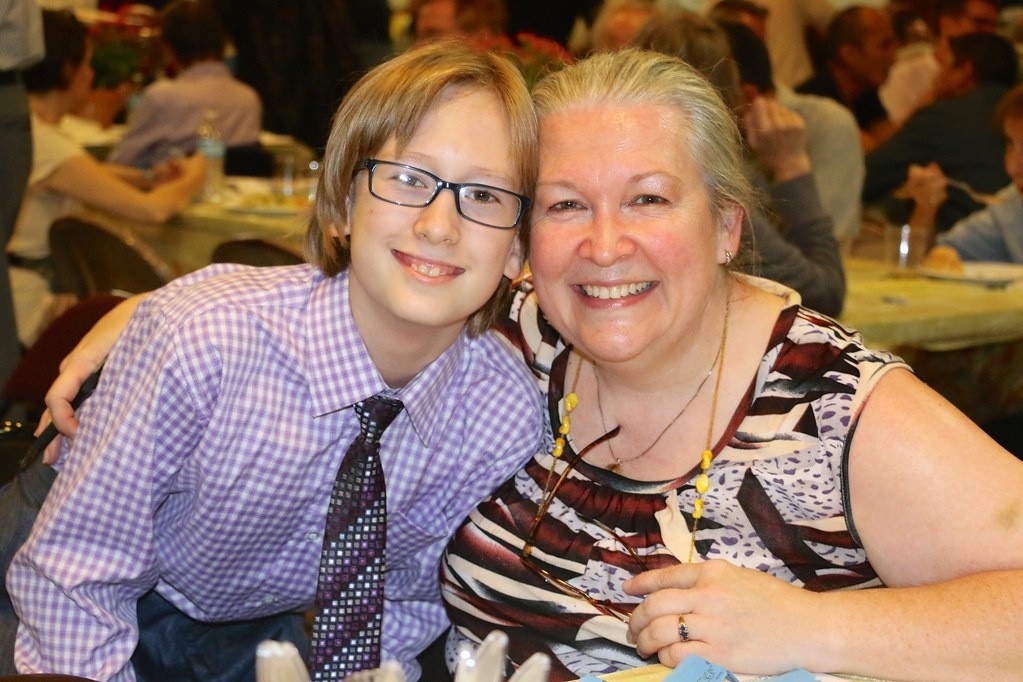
[268,155,298,204]
[881,224,926,277]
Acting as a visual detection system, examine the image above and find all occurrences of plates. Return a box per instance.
[223,200,306,220]
[917,259,1022,291]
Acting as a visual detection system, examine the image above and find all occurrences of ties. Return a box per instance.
[306,395,405,681]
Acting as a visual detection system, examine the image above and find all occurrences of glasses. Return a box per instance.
[353,156,532,231]
[519,424,699,625]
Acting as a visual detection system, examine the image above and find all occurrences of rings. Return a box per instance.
[679,615,692,642]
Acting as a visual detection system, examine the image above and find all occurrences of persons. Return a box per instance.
[593,1,653,51]
[626,11,845,318]
[414,1,514,67]
[32,50,1021,680]
[858,31,1018,221]
[0,0,47,388]
[104,3,260,171]
[9,7,205,342]
[894,14,938,45]
[711,0,767,35]
[702,20,862,261]
[792,8,936,154]
[905,88,1023,265]
[5,39,544,682]
[881,1,1001,120]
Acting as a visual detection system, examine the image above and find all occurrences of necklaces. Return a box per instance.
[591,334,723,471]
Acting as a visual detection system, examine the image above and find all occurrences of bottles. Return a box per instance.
[193,110,226,204]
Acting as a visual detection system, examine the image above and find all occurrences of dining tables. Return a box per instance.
[161,172,1022,349]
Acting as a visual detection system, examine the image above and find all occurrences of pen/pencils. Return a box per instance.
[19,354,110,472]
